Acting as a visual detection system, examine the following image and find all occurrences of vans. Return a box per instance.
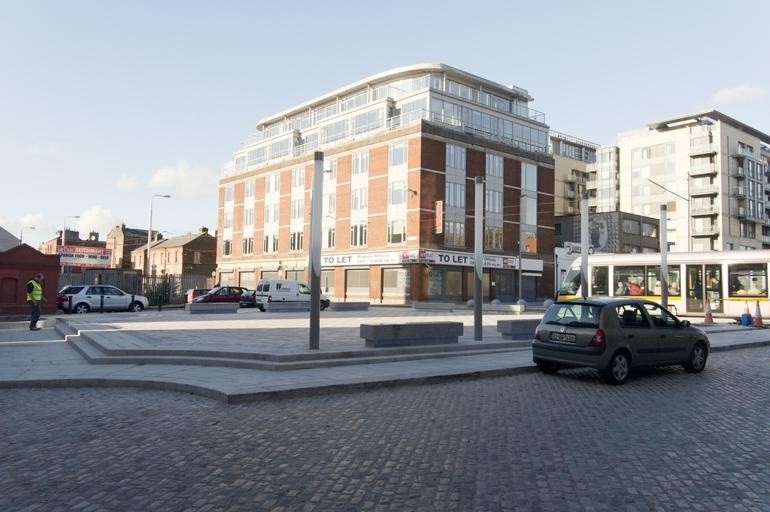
[256,279,330,311]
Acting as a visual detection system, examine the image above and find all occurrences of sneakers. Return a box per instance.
[30,327,41,331]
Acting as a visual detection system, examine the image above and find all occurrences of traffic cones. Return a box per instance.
[742,298,751,314]
[752,298,765,328]
[703,297,716,325]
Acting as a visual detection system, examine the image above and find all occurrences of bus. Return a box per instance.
[553,249,770,324]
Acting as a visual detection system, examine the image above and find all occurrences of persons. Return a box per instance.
[25,272,49,332]
[593,272,765,302]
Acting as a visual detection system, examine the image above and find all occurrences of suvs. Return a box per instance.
[56,284,149,313]
[533,292,710,385]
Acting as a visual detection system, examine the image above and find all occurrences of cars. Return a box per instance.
[183,285,256,307]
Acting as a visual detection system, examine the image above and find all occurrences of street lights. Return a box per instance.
[60,216,81,270]
[472,176,485,340]
[579,192,590,317]
[309,150,324,348]
[146,194,173,293]
[660,204,670,319]
[20,226,36,246]
